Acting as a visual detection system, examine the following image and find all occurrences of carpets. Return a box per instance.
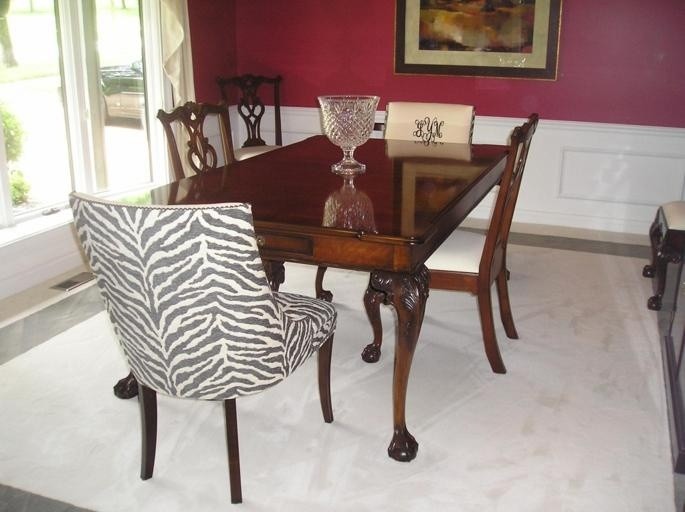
[0,229,683,510]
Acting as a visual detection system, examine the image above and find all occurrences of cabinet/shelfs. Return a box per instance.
[658,261,685,475]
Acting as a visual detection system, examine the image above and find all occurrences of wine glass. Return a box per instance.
[316,94,379,175]
[321,175,379,238]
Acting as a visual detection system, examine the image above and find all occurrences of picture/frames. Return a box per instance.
[392,0,565,82]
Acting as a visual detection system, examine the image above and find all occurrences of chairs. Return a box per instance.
[639,197,685,311]
[314,78,481,302]
[215,73,283,167]
[359,109,542,376]
[155,98,236,184]
[70,190,339,505]
